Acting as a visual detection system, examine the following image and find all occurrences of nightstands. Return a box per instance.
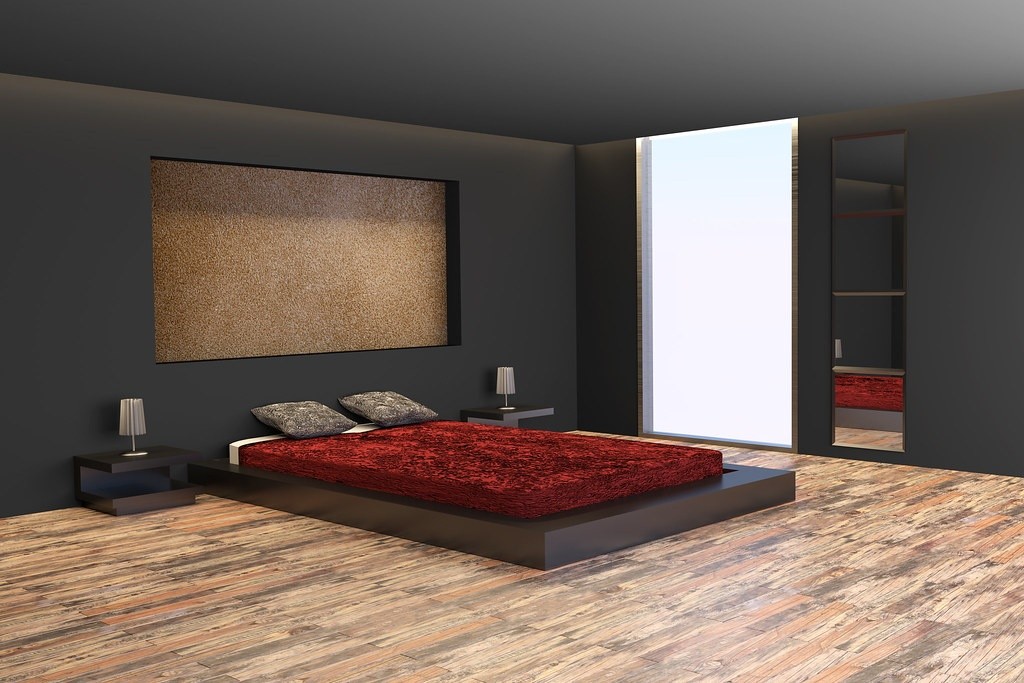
[460,405,554,428]
[73,444,203,515]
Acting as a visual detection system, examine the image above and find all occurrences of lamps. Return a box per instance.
[835,339,842,365]
[496,367,516,409]
[119,397,150,457]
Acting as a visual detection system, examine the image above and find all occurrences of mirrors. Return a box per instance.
[831,129,909,453]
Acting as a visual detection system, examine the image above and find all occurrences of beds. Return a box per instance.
[833,373,904,433]
[186,418,796,571]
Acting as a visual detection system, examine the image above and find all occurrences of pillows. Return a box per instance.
[250,400,357,440]
[337,390,438,428]
